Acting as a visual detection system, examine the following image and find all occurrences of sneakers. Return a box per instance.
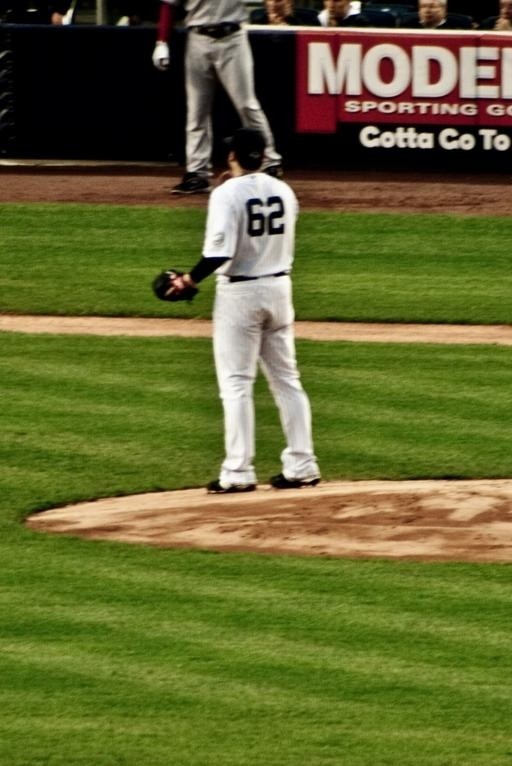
[265,164,284,180]
[270,473,320,488]
[169,173,214,194]
[207,478,256,493]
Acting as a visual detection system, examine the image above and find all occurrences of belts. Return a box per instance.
[229,271,286,282]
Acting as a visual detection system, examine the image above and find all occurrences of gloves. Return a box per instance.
[152,41,170,70]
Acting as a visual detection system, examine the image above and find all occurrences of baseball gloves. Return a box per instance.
[152,269,199,301]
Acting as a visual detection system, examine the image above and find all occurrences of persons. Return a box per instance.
[166,127,322,494]
[478,0,512,31]
[317,0,370,27]
[0,0,76,26]
[250,0,309,26]
[170,0,284,196]
[116,11,146,27]
[398,0,472,30]
